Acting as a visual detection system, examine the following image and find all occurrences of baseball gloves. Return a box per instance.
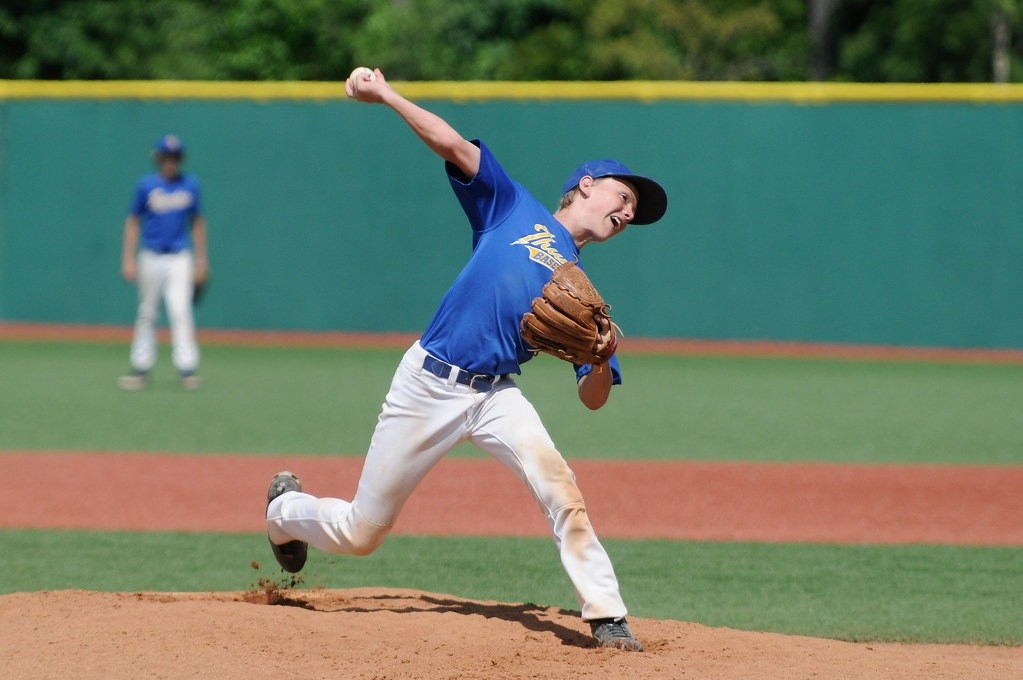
[517,253,625,376]
[194,282,205,305]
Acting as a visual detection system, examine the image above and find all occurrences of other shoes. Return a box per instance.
[117,373,147,389]
[182,377,199,391]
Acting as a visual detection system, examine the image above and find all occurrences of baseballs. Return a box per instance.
[350,66,377,82]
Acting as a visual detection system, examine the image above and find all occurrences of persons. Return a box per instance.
[116,132,209,384]
[263,67,668,653]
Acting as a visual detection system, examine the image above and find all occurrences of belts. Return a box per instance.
[422,355,509,392]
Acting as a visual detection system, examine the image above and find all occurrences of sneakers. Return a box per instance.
[265,472,308,573]
[590,617,643,651]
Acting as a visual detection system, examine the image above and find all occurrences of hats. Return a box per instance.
[563,159,668,226]
[153,132,184,156]
